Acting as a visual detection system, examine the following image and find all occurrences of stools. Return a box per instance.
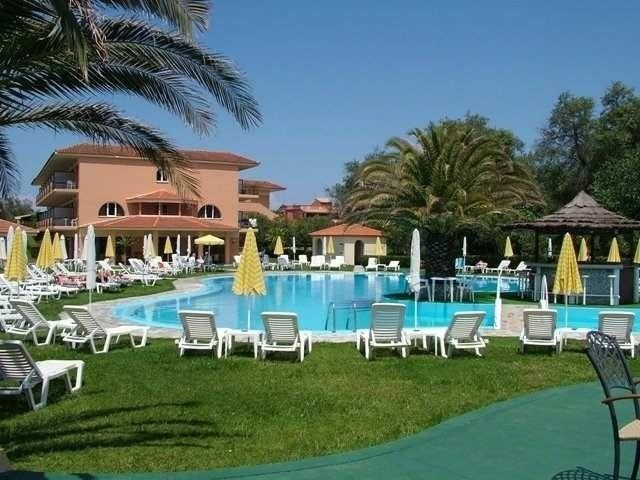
[531,274,616,306]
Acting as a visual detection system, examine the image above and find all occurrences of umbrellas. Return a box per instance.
[1,222,225,314]
[230,226,267,350]
[273,228,467,332]
[502,229,640,327]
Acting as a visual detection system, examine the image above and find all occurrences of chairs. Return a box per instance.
[463,259,529,276]
[0,255,203,333]
[519,309,563,354]
[355,303,410,359]
[581,331,640,479]
[259,312,312,362]
[408,310,486,356]
[0,339,84,412]
[173,308,226,360]
[6,299,73,345]
[590,310,636,359]
[403,270,476,303]
[62,304,151,354]
[232,252,401,272]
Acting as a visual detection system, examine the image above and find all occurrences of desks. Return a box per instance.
[224,328,266,360]
